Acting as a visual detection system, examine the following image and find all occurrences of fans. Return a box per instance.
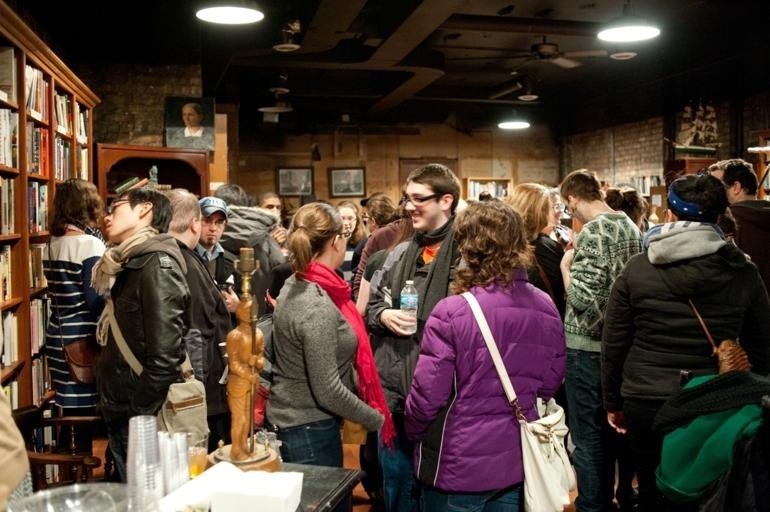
[425,8,609,68]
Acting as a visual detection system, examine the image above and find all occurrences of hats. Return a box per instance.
[199,197,228,222]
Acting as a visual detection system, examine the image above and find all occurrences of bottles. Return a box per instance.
[400,280,419,333]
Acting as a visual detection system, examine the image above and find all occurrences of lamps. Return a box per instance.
[595,0,663,67]
[191,0,265,25]
[257,94,294,114]
[498,107,531,134]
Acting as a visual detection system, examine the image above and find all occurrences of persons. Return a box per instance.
[43,159,770,511]
[171,103,213,152]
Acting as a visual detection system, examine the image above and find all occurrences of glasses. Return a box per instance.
[361,212,372,220]
[402,195,436,203]
[340,231,351,239]
[108,199,145,214]
[564,200,571,214]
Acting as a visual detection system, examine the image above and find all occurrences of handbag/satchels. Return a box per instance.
[64,336,100,384]
[157,381,210,447]
[520,398,578,512]
[714,340,750,374]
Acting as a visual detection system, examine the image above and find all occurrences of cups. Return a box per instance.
[188,433,209,478]
[127,415,188,498]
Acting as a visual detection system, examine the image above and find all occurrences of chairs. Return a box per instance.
[10,405,103,491]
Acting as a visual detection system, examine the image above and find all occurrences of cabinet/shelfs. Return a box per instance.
[0,1,104,410]
[461,177,514,201]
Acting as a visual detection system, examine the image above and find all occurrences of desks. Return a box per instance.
[281,461,363,512]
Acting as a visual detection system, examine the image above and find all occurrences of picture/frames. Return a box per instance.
[330,168,366,198]
[275,168,313,198]
[163,96,216,154]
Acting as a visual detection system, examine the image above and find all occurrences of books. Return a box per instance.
[1,43,90,484]
